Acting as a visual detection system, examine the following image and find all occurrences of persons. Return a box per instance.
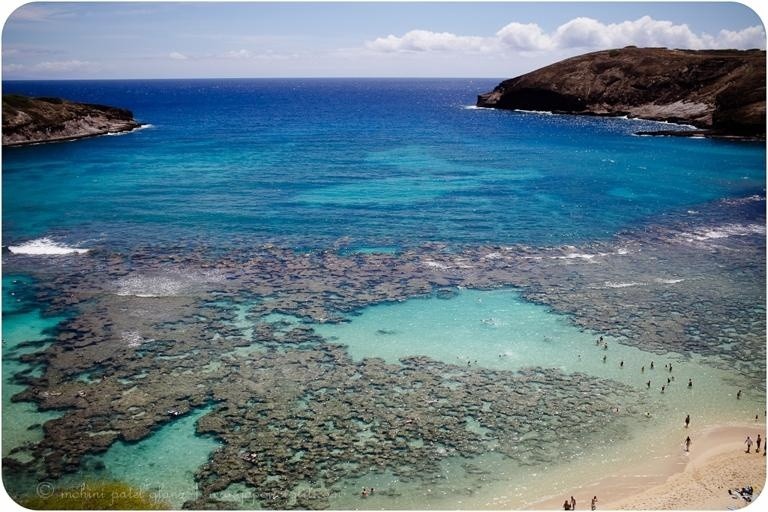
[591,496,598,511]
[578,336,766,454]
[361,488,374,496]
[563,495,577,511]
[728,486,753,502]
